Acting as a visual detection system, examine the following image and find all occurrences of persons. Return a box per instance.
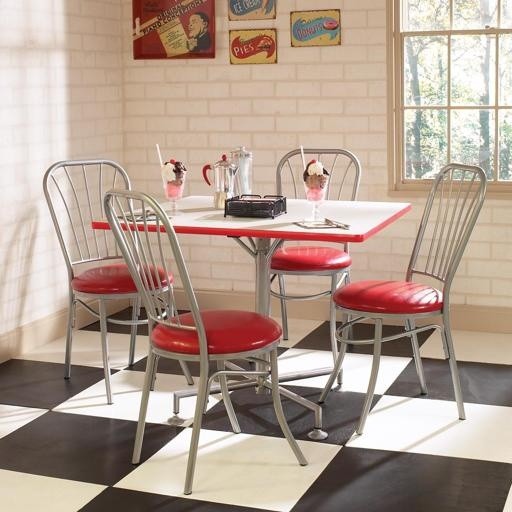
[184,12,212,54]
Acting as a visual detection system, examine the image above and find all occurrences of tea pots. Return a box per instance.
[202,154,240,209]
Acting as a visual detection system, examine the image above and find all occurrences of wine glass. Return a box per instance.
[303,174,330,222]
[160,164,187,217]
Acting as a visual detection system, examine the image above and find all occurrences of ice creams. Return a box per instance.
[303,160,329,201]
[160,160,186,199]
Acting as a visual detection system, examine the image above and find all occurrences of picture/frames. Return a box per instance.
[132,0,215,60]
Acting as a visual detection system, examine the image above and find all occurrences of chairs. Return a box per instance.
[105,189,309,495]
[43,160,197,406]
[318,162,488,433]
[268,148,363,383]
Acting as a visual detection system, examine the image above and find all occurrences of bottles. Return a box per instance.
[229,146,252,196]
